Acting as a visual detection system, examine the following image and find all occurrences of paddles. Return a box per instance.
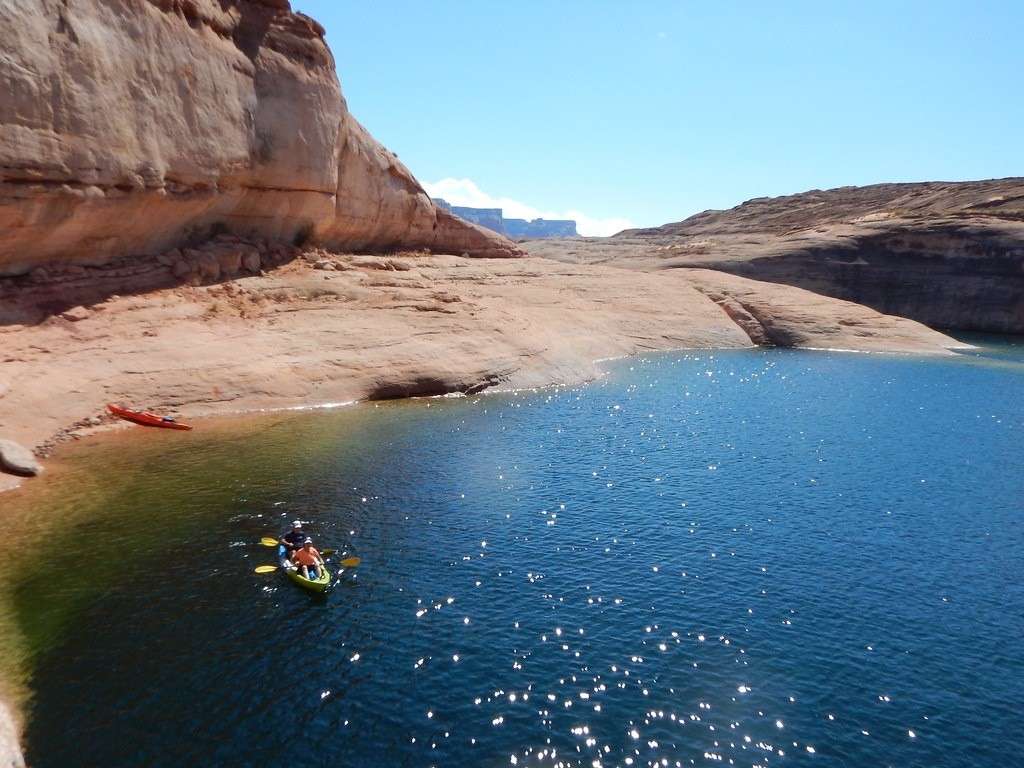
[255,557,363,573]
[260,537,335,552]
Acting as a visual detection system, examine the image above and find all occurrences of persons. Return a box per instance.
[292,537,325,581]
[279,521,306,565]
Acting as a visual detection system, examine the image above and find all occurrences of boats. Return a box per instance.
[277,543,330,591]
[108,403,193,430]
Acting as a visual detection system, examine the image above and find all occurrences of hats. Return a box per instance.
[303,537,312,544]
[293,520,302,528]
